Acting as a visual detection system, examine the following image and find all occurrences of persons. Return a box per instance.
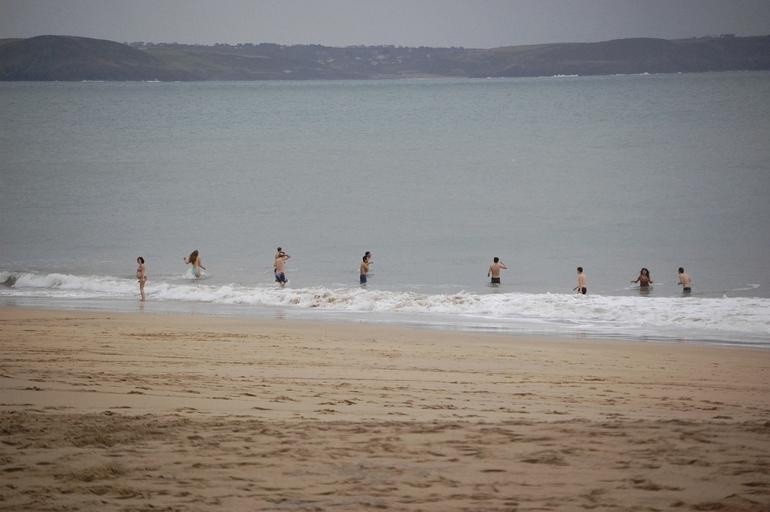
[183,249,206,278]
[136,256,147,301]
[365,251,372,258]
[360,256,374,284]
[274,247,282,272]
[573,267,587,294]
[677,267,691,294]
[275,251,290,283]
[630,268,653,287]
[488,257,507,284]
